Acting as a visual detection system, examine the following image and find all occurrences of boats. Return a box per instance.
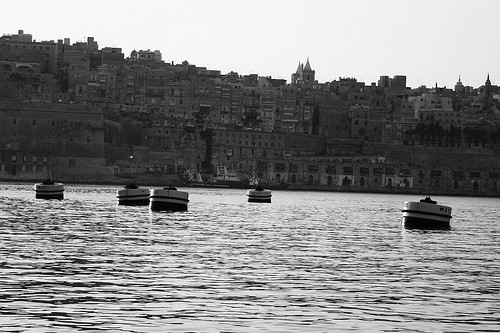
[401,196,453,230]
[247,185,272,203]
[149,186,189,213]
[178,165,258,187]
[116,182,151,206]
[34,178,65,201]
